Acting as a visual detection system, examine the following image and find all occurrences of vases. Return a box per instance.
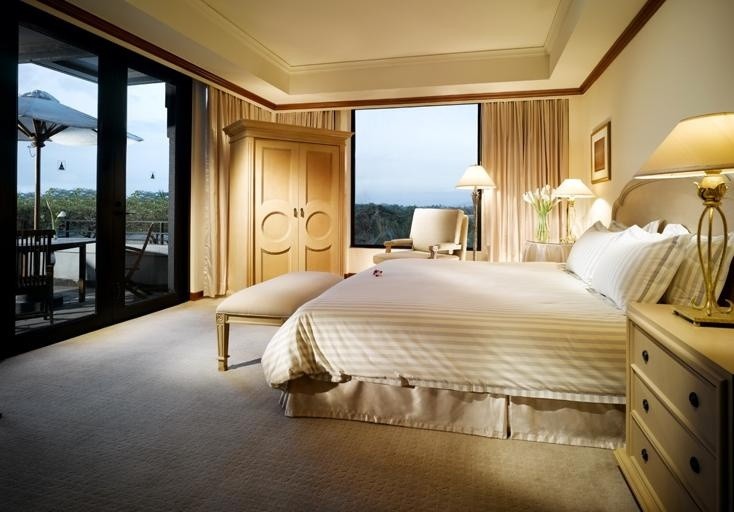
[536,213,551,243]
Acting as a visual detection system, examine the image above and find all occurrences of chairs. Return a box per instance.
[373,208,468,264]
[14,229,56,325]
[125,223,154,298]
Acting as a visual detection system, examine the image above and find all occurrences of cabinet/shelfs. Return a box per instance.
[222,119,354,296]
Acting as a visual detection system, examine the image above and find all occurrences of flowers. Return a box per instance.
[522,185,561,214]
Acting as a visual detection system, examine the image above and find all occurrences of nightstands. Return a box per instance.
[523,240,573,262]
[614,303,734,512]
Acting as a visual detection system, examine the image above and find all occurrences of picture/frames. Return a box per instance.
[591,121,611,184]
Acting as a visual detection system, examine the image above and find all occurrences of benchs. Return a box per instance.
[216,271,344,371]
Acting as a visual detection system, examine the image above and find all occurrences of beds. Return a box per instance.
[287,173,734,447]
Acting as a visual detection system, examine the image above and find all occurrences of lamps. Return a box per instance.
[455,165,497,261]
[635,111,734,328]
[552,179,596,243]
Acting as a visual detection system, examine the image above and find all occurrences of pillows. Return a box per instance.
[565,221,688,314]
[661,221,734,312]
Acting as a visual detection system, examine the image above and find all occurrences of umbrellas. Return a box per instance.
[18,90,142,274]
